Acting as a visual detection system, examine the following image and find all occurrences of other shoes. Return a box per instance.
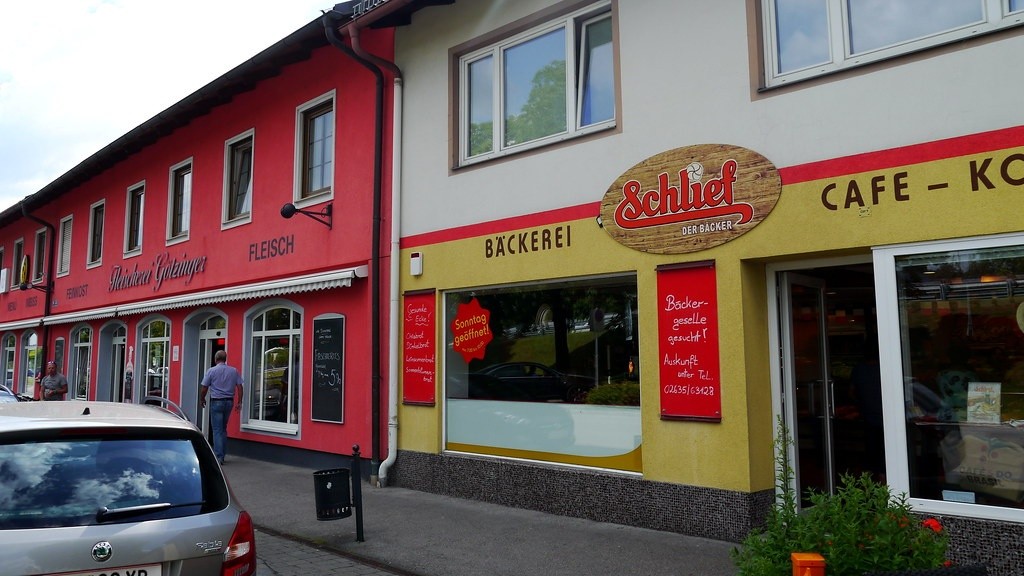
[217,456,224,465]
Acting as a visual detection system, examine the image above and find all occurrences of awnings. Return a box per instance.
[43,306,117,326]
[116,264,369,317]
[0,317,43,331]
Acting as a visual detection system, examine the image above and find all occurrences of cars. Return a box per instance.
[6,369,12,386]
[254,366,288,408]
[450,362,601,404]
[795,376,966,495]
[264,347,286,361]
[144,367,168,406]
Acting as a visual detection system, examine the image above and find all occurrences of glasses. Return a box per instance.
[47,360,56,364]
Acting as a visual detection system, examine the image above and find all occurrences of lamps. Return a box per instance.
[20,281,55,293]
[279,202,332,230]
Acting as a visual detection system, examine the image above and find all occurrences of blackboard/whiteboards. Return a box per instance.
[309,312,345,424]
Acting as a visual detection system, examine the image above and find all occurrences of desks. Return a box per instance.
[907,423,1024,509]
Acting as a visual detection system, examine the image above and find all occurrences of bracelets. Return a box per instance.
[54,390,57,394]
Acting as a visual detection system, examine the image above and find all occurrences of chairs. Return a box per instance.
[95,440,146,501]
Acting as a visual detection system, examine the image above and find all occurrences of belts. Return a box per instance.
[211,398,233,401]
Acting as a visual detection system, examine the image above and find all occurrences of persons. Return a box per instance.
[39,361,68,401]
[200,350,244,465]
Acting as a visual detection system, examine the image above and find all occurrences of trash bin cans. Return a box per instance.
[313,467,351,521]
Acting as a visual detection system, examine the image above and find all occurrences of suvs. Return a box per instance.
[0,396,257,576]
[0,383,21,403]
